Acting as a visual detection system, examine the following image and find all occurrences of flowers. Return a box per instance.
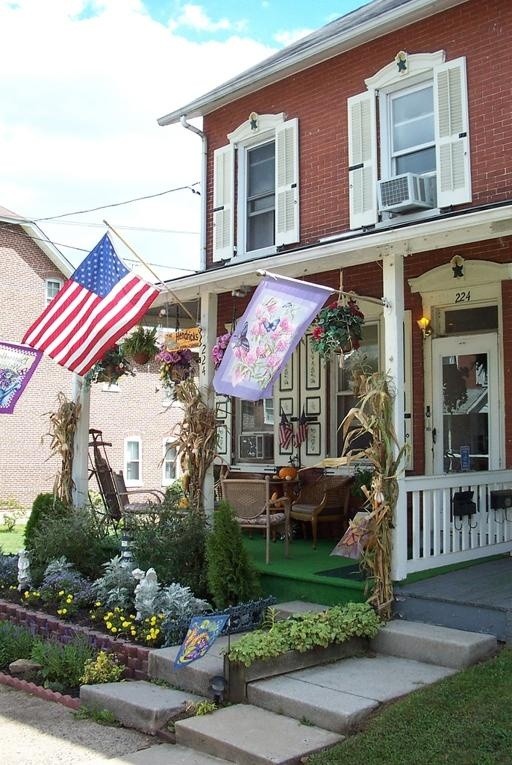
[312,300,366,353]
[155,344,201,389]
[211,334,231,370]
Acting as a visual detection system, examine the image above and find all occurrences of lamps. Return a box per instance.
[417,317,432,339]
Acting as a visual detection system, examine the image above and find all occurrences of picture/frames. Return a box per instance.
[278,333,321,456]
[216,402,227,419]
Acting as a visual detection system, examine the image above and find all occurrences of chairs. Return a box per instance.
[88,428,356,566]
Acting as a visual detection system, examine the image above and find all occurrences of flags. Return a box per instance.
[0,343,43,414]
[174,613,231,669]
[20,233,162,376]
[212,272,335,402]
[330,512,374,560]
[280,423,293,449]
[294,422,308,448]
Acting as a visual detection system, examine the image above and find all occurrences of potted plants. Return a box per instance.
[96,343,136,388]
[220,602,386,703]
[119,326,161,365]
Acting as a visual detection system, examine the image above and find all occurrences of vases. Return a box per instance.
[334,338,359,352]
[170,366,185,381]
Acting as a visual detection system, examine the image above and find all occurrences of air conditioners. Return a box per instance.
[376,172,432,214]
